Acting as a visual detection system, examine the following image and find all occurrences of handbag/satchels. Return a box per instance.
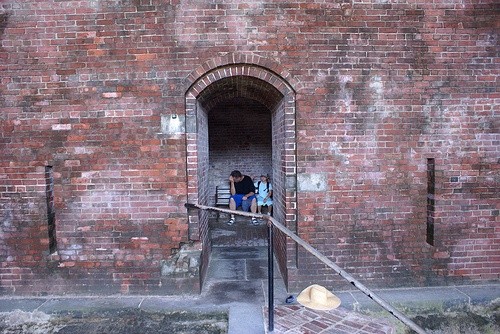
[241,199,250,212]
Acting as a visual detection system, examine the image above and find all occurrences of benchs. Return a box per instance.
[214,184,262,221]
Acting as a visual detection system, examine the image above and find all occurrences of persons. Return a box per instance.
[227,170,259,226]
[253,172,273,221]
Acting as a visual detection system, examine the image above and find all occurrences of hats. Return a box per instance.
[260,172,268,178]
[296,284,342,311]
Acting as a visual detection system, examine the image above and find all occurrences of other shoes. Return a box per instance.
[251,218,259,225]
[226,219,236,226]
